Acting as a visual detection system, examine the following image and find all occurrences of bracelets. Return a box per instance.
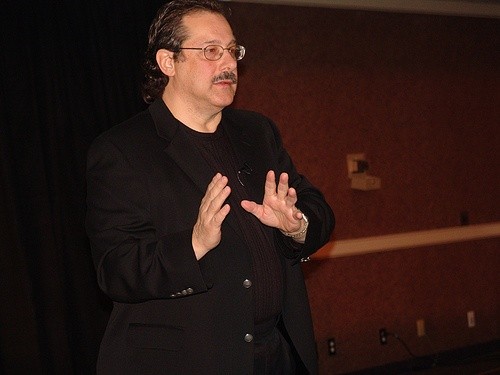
[280,211,310,239]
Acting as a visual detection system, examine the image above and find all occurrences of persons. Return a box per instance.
[85,2,335,373]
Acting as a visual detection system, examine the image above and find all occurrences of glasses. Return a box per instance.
[181,45,246,62]
